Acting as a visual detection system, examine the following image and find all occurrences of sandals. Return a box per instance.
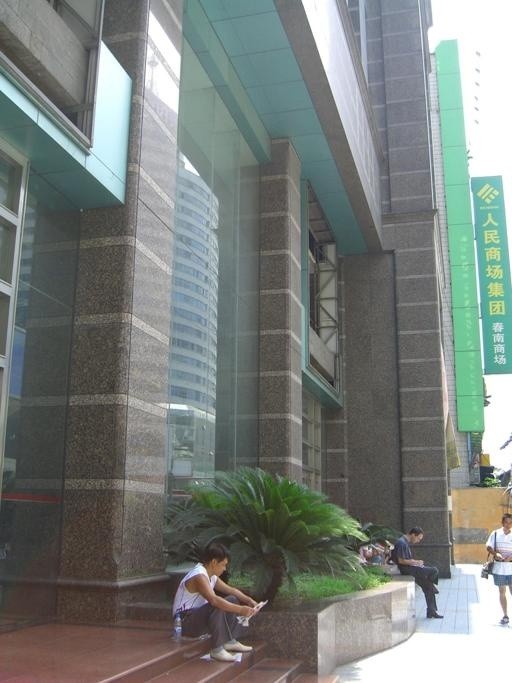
[500,617,509,624]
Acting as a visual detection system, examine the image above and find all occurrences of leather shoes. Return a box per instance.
[211,649,237,662]
[224,641,253,652]
[423,583,439,593]
[427,613,444,618]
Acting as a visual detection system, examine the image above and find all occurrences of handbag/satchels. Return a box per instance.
[481,560,493,578]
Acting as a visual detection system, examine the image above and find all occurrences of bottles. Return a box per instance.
[173,614,182,640]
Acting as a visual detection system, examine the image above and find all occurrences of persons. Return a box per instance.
[172,543,261,660]
[486,513,512,624]
[392,529,445,618]
